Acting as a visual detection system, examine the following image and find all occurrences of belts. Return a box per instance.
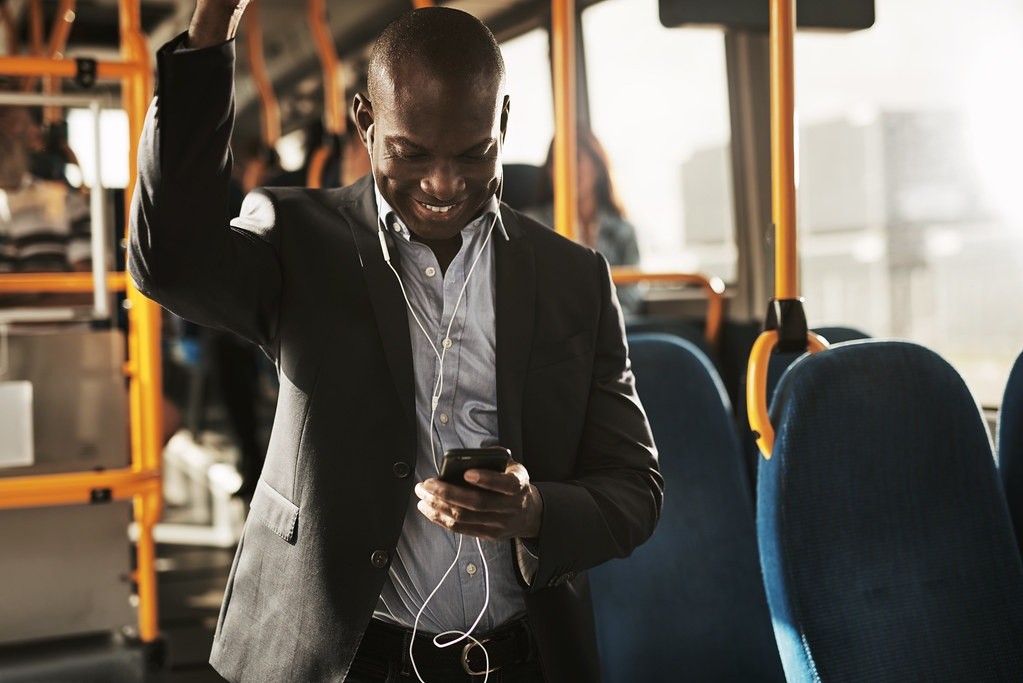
[358,627,529,676]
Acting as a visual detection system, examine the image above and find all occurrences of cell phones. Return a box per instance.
[436,447,512,494]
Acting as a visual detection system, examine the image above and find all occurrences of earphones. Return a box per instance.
[366,124,375,156]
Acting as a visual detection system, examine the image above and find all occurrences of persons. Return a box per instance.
[126,0,667,683]
[0,107,96,307]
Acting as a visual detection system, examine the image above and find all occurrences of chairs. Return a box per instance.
[999,351,1023,558]
[577,334,786,683]
[730,325,873,485]
[756,341,1023,683]
